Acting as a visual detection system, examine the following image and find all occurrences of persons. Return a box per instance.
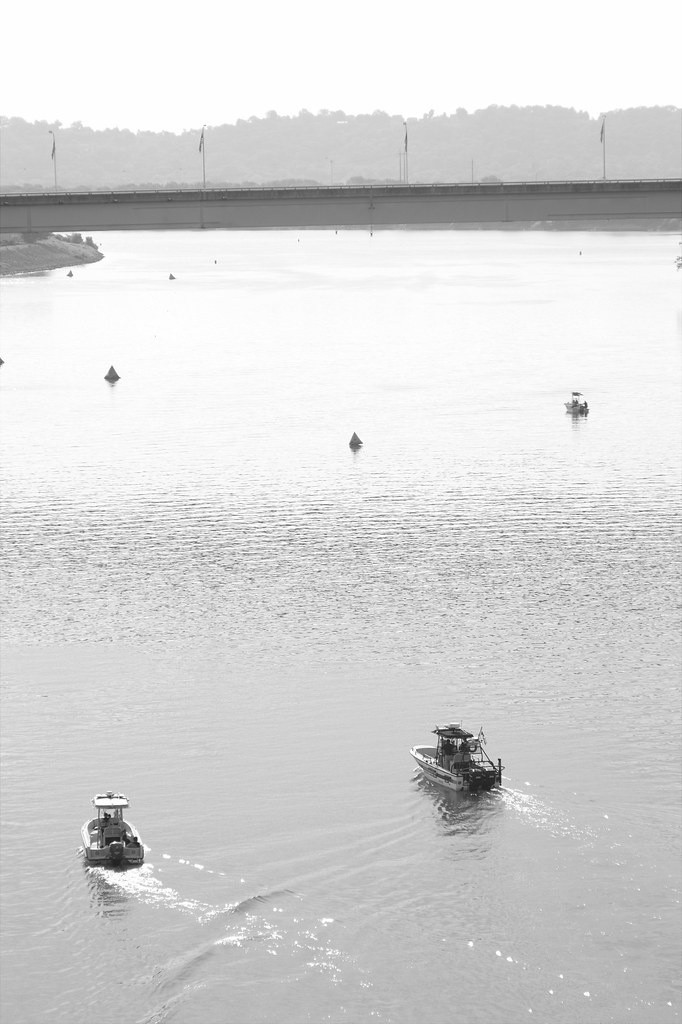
[100,809,140,848]
[442,738,469,768]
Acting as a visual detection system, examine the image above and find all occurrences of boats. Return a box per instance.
[78,790,144,865]
[104,365,122,381]
[349,430,364,446]
[168,274,176,280]
[409,720,508,794]
[0,358,6,369]
[564,390,590,415]
[65,270,74,277]
